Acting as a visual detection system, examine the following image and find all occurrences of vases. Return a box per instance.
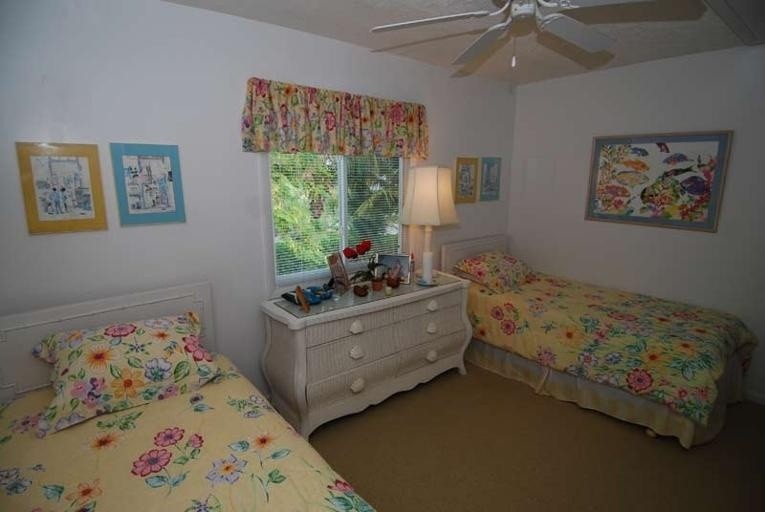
[371,279,384,291]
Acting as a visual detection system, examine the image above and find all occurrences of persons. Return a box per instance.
[50,187,64,215]
[58,187,69,214]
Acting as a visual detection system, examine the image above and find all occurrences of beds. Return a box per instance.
[0,282,379,512]
[439,233,742,438]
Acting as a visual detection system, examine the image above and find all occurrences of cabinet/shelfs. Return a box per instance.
[260,266,474,442]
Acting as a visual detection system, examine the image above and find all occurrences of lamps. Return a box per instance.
[399,166,461,286]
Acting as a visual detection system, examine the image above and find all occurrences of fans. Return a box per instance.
[369,0,620,73]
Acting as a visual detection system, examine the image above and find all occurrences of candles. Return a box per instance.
[422,251,434,283]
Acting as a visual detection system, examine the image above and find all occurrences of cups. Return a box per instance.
[385,286,392,295]
[372,278,383,291]
[332,288,340,301]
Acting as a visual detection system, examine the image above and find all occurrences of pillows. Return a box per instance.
[28,320,226,438]
[452,251,531,294]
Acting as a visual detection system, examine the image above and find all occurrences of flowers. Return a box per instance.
[342,240,388,284]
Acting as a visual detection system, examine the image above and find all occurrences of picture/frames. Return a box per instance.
[454,156,479,204]
[108,141,188,227]
[16,141,110,236]
[480,157,501,201]
[584,130,733,232]
[327,253,351,296]
[375,253,412,285]
